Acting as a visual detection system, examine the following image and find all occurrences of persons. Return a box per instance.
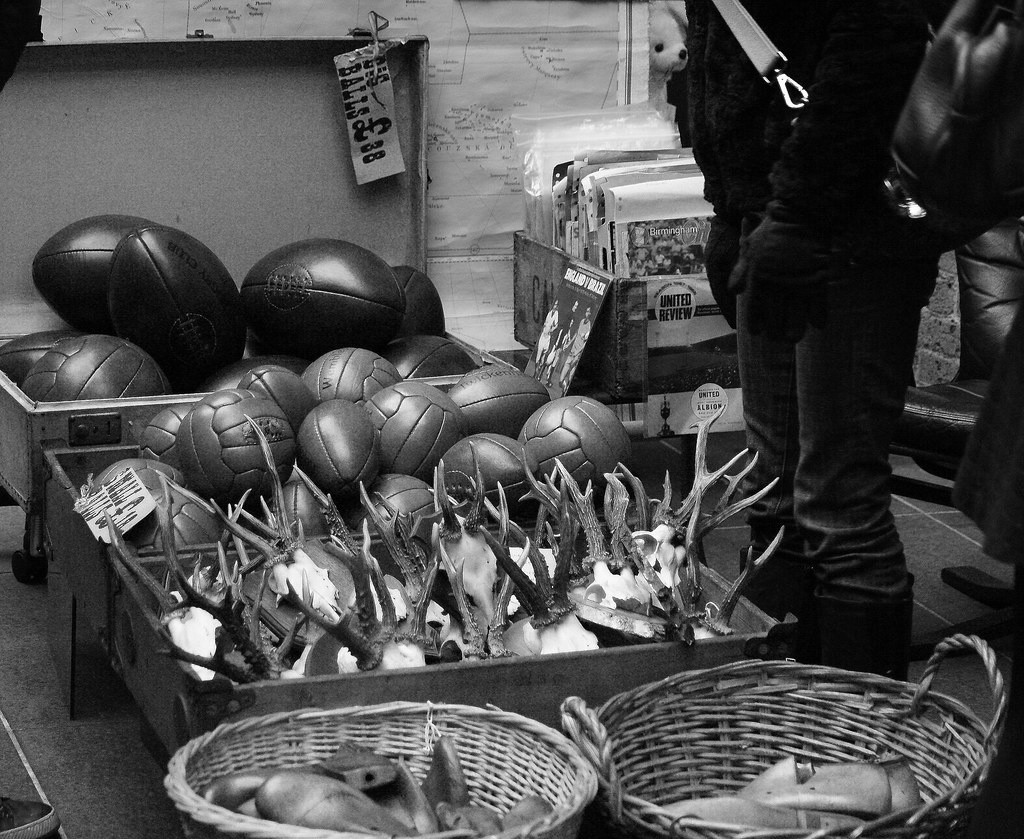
[677,0,929,686]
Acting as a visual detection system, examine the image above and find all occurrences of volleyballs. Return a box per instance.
[297,345,404,405]
[140,403,192,468]
[180,387,295,515]
[133,489,222,574]
[365,385,467,478]
[518,395,633,504]
[345,472,448,537]
[91,458,184,492]
[23,337,177,400]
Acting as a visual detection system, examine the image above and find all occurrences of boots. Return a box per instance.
[739,547,820,664]
[813,571,916,683]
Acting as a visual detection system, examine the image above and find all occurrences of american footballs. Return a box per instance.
[300,403,378,494]
[238,236,410,359]
[0,329,74,380]
[451,371,551,434]
[109,226,249,387]
[266,479,332,538]
[438,432,542,513]
[237,365,316,428]
[27,212,167,324]
[396,334,480,376]
[392,265,446,331]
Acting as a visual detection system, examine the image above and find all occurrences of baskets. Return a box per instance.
[558,629,1012,839]
[162,697,600,839]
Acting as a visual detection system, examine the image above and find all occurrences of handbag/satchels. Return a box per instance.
[869,1,1024,263]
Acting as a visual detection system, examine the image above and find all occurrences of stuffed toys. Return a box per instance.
[647,7,690,103]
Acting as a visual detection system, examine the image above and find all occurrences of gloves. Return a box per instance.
[725,217,827,346]
[704,217,743,329]
